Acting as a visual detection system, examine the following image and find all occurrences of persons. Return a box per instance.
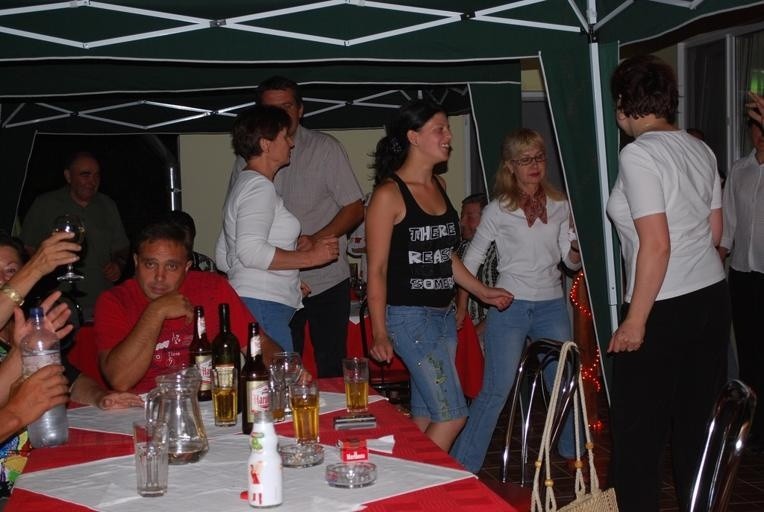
[20,149,136,387]
[457,191,499,353]
[0,231,146,412]
[214,105,340,367]
[120,212,217,283]
[93,211,312,414]
[223,73,366,379]
[0,290,73,446]
[449,130,604,477]
[717,91,764,433]
[366,99,512,452]
[605,58,731,512]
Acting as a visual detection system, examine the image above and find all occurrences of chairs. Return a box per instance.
[689,376,758,512]
[484,334,582,508]
[358,294,412,407]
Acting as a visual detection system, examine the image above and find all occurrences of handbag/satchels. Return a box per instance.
[555,489,618,512]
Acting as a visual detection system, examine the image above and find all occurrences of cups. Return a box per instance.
[132,418,169,497]
[211,368,238,426]
[289,380,321,445]
[342,356,372,413]
[268,365,286,424]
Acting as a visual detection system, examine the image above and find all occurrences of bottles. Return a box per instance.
[147,368,209,465]
[247,413,282,508]
[188,305,213,402]
[211,302,240,414]
[240,321,273,435]
[20,306,70,451]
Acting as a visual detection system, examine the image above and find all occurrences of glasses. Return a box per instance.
[510,154,547,165]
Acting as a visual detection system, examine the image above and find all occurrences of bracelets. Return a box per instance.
[2,282,25,307]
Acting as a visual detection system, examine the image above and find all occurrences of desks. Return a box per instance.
[1,375,517,512]
[68,289,482,398]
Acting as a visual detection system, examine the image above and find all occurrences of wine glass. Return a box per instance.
[51,210,86,283]
[272,350,303,415]
